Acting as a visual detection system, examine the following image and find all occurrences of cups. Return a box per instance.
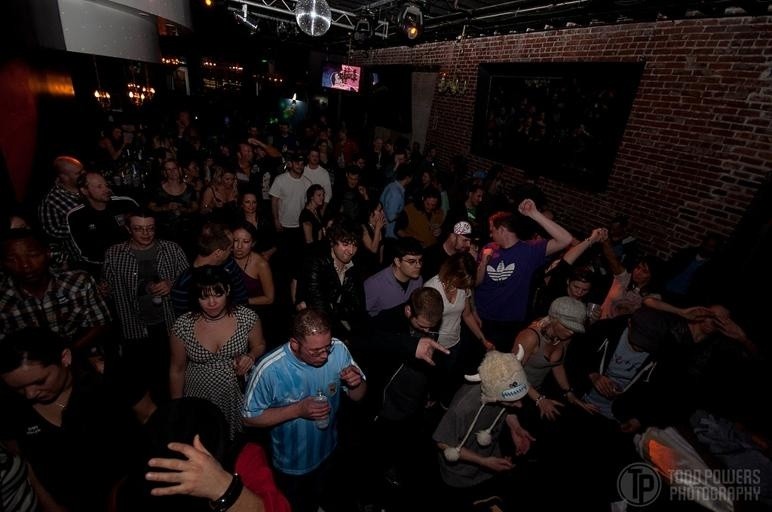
[152,283,163,307]
[432,224,440,239]
[114,176,120,186]
[586,302,601,319]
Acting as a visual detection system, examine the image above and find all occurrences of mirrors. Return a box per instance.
[471,62,644,192]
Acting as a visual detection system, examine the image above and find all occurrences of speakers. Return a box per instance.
[351,30,369,49]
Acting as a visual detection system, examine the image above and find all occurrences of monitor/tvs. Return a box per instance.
[321,63,363,95]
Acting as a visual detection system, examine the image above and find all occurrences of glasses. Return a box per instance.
[298,339,335,356]
[133,226,155,232]
[401,258,424,264]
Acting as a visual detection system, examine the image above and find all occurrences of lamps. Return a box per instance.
[438,25,467,97]
[126,65,156,107]
[388,4,423,39]
[234,11,259,31]
[91,60,111,111]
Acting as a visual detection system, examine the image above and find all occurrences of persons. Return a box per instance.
[1,104,772,511]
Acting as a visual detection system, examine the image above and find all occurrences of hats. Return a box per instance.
[628,307,662,352]
[449,221,477,238]
[285,152,304,160]
[465,344,529,404]
[548,296,587,334]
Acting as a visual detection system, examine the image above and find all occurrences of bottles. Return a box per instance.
[315,388,330,430]
[234,351,250,394]
[121,144,152,190]
